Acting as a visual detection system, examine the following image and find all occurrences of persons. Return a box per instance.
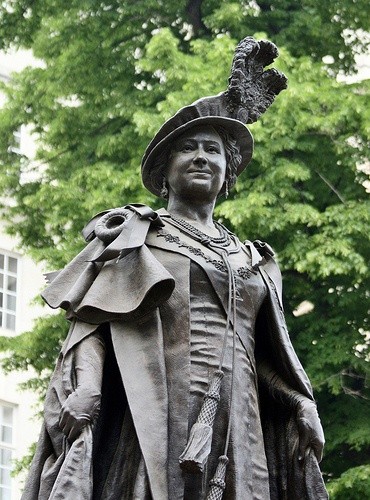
[20,105,330,500]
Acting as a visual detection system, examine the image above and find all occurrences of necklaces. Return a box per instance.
[167,210,232,246]
